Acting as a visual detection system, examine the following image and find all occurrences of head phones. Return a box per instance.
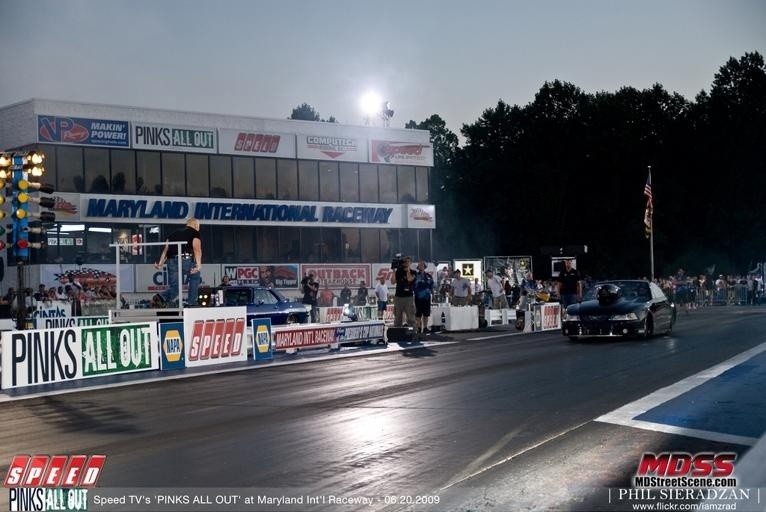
[416,262,427,270]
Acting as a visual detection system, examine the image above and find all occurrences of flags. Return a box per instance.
[644,173,656,239]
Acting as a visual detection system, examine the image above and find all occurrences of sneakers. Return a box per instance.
[153,295,166,307]
[423,328,431,333]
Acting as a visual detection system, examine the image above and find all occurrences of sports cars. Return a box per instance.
[559,278,676,343]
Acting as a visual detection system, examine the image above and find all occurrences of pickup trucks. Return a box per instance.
[215,283,308,329]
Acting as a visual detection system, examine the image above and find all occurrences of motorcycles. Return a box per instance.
[514,287,546,330]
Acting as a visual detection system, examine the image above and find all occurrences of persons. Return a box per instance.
[2,271,129,319]
[152,216,204,307]
[300,272,319,322]
[220,276,232,286]
